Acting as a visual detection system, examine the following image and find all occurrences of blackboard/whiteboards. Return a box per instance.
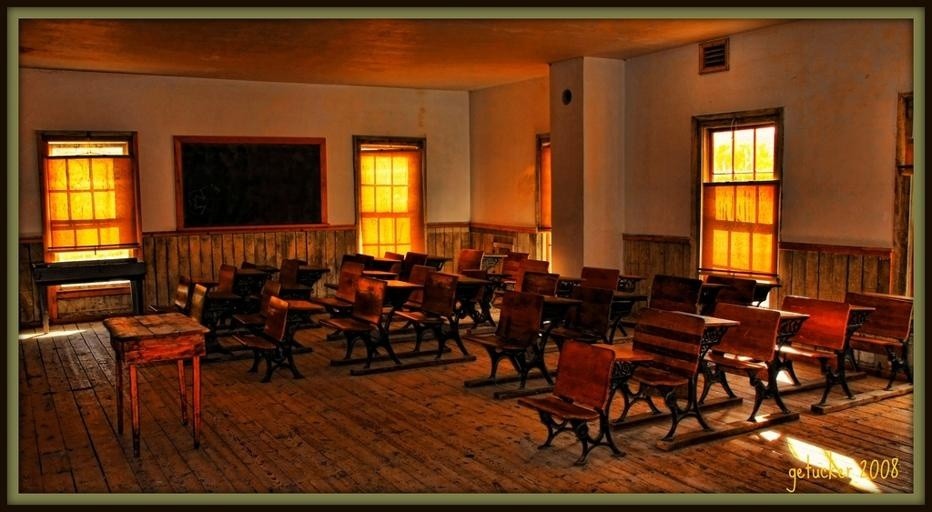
[174,135,330,231]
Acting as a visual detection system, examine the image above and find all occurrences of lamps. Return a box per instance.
[20,209,43,325]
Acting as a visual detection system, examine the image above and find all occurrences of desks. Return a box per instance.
[103,312,210,460]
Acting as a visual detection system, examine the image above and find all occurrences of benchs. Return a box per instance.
[148,246,915,466]
